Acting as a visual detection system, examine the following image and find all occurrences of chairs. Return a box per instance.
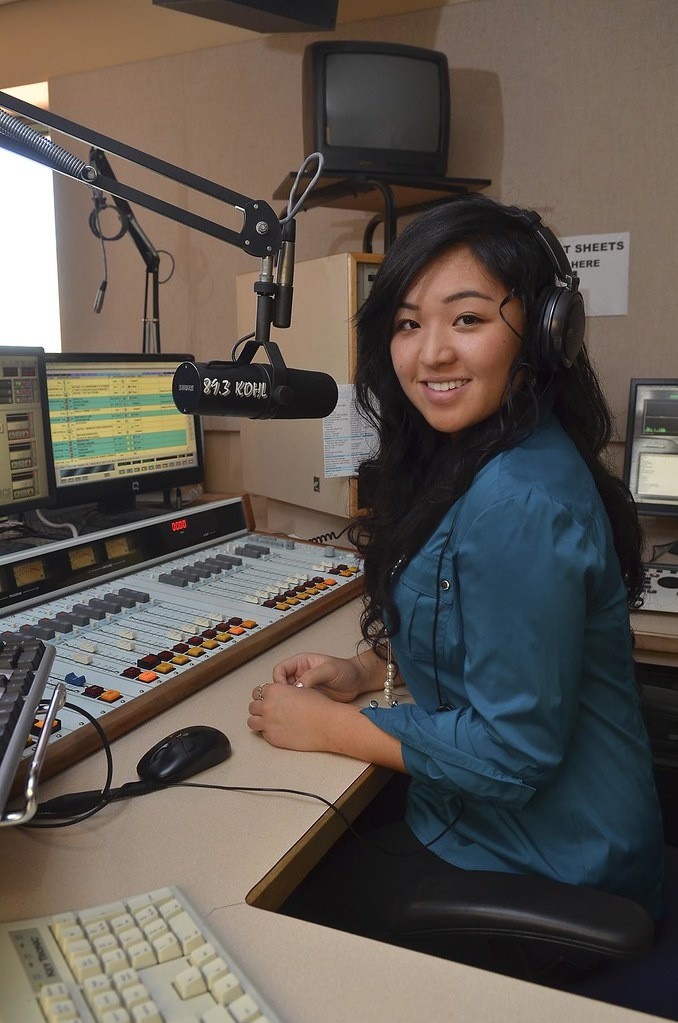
[359,667,678,1017]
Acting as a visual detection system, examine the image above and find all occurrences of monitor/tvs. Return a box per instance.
[0,345,204,517]
[302,40,451,177]
[622,378,678,517]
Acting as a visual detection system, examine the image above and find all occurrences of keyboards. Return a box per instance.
[0,639,57,817]
[0,885,283,1023]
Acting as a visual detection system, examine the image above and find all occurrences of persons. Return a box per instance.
[248,198,669,987]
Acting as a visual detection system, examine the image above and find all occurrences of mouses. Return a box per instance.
[137,724,231,785]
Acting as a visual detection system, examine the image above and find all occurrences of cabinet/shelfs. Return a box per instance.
[243,170,494,519]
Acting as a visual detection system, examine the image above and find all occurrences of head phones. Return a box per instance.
[498,203,586,372]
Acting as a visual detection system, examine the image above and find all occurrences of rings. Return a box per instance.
[257,683,268,700]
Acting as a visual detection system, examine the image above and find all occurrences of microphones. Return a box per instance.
[173,359,339,420]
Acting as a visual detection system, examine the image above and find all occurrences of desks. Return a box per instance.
[2,510,677,1023]
[624,508,677,664]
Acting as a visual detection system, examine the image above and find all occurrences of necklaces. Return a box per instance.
[383,553,408,708]
